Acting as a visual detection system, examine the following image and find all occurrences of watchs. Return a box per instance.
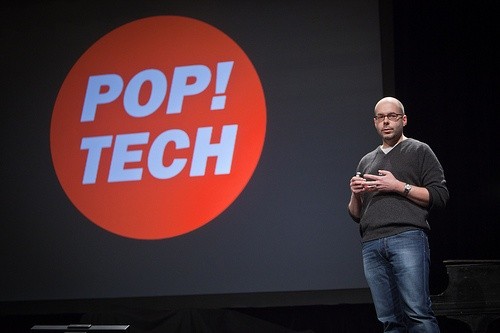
[401,183,413,197]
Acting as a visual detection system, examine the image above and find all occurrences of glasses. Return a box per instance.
[374,113,403,121]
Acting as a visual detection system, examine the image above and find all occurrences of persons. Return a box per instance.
[347,97,449,333]
